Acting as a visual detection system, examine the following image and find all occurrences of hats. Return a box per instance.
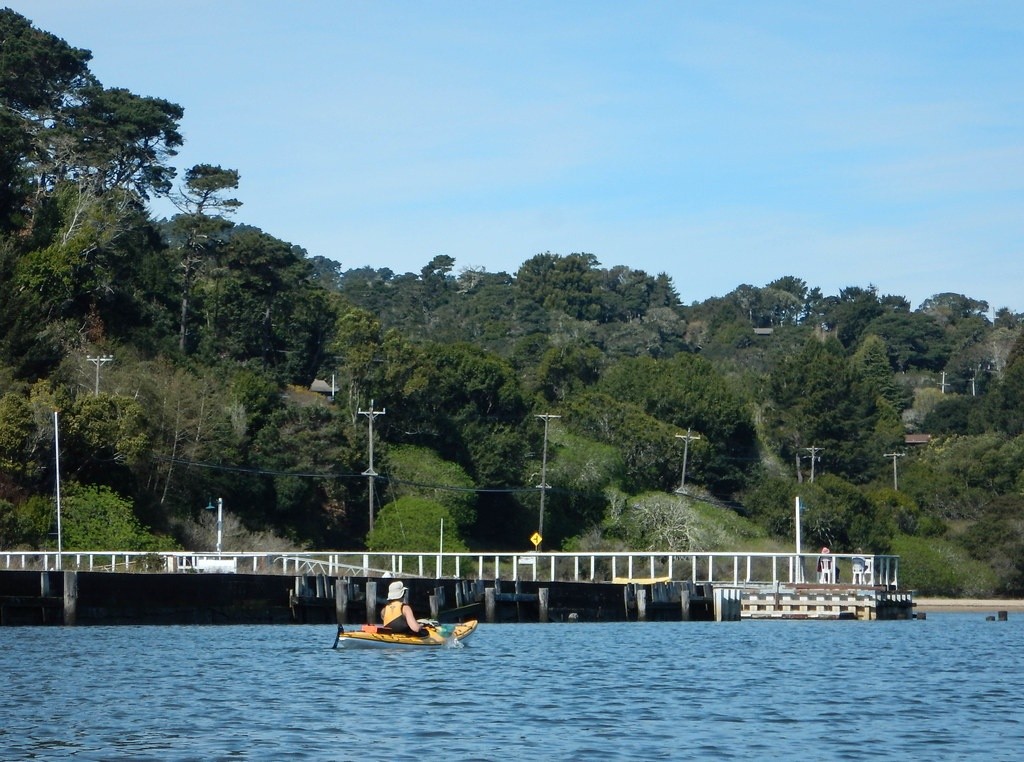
[386,581,409,601]
[822,547,830,553]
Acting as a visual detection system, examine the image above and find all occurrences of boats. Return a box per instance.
[332,619,478,651]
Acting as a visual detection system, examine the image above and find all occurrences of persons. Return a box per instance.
[816,547,840,584]
[381,581,425,633]
[850,548,868,573]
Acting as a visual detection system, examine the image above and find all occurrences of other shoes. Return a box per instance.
[836,580,840,583]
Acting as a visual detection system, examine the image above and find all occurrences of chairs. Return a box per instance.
[863,559,875,585]
[851,557,867,585]
[819,558,832,584]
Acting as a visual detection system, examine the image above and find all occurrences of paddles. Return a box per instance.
[430,623,453,637]
[423,627,445,642]
